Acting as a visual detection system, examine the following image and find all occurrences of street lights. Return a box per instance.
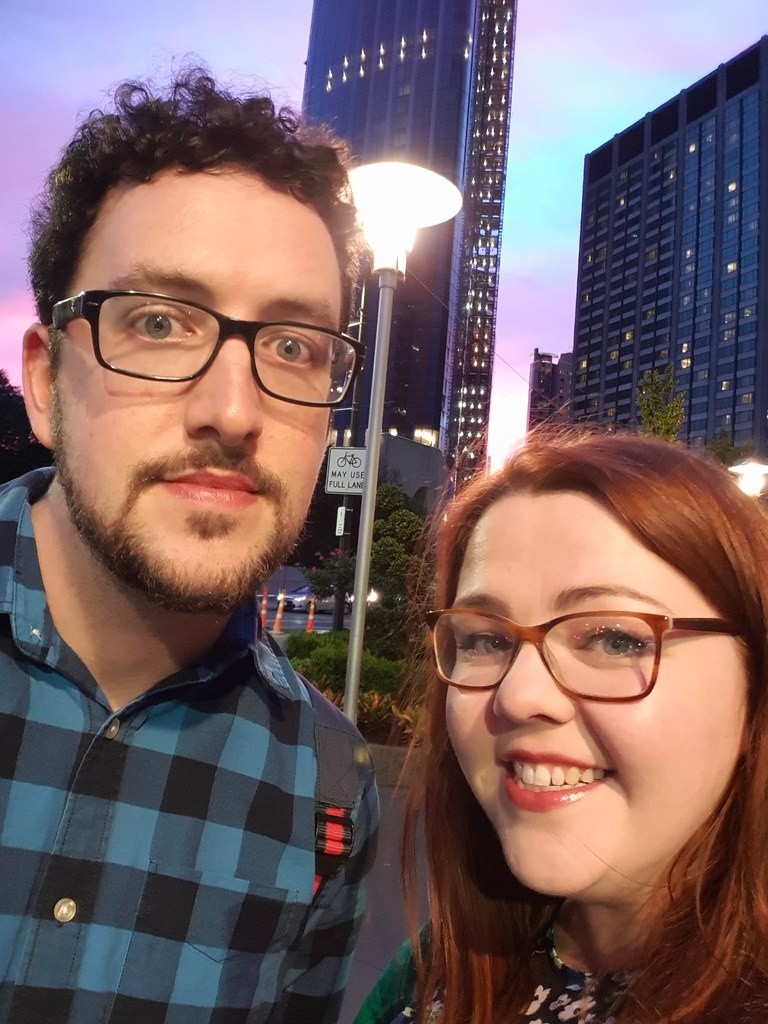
[336,158,464,726]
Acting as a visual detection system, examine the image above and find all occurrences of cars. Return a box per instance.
[278,585,351,615]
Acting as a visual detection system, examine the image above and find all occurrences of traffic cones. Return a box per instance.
[260,587,267,629]
[306,599,315,633]
[273,589,287,633]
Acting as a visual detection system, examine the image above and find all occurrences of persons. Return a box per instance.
[351,432,768,1024]
[0,67,382,1024]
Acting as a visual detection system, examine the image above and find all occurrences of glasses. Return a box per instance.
[51,289,368,409]
[424,607,742,703]
